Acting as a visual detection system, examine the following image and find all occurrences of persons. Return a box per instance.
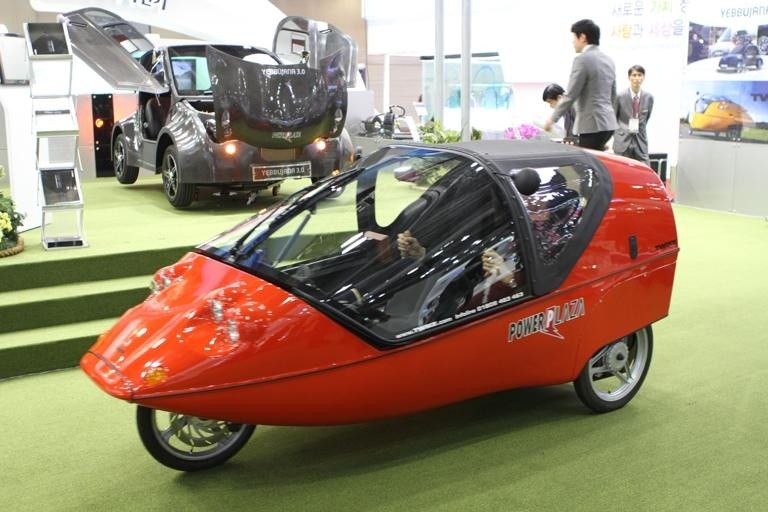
[395,190,573,314]
[613,65,653,169]
[543,83,575,140]
[543,18,618,150]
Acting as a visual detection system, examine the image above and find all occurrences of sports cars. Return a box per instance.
[54,7,361,206]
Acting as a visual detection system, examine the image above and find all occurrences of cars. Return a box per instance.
[718,44,764,71]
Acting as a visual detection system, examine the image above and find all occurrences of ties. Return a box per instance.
[631,94,641,119]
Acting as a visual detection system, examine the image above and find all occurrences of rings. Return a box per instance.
[397,244,400,246]
[486,257,491,262]
